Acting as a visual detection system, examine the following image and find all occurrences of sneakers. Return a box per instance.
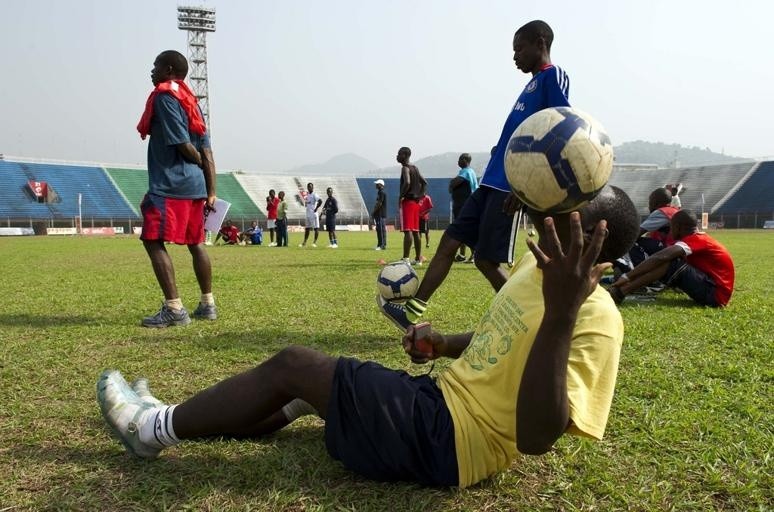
[376,247,385,250]
[376,293,416,334]
[225,242,231,245]
[276,244,281,246]
[209,242,214,246]
[243,242,246,245]
[426,244,429,247]
[464,258,474,263]
[399,258,410,266]
[269,242,276,247]
[142,301,191,328]
[190,302,217,320]
[411,260,423,266]
[239,242,242,245]
[454,255,466,262]
[607,286,624,305]
[284,243,288,246]
[300,244,306,247]
[311,244,316,247]
[328,244,333,248]
[333,244,338,248]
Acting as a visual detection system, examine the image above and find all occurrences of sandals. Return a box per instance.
[133,378,165,412]
[98,368,163,461]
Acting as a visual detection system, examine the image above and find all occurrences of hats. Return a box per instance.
[374,179,384,185]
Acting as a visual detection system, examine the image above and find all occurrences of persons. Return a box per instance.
[203,182,340,250]
[418,192,434,248]
[396,147,428,266]
[136,49,219,326]
[598,184,736,309]
[376,19,570,336]
[447,153,479,263]
[368,179,387,250]
[94,183,641,489]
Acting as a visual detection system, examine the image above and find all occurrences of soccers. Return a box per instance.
[504,105,614,212]
[378,260,420,303]
